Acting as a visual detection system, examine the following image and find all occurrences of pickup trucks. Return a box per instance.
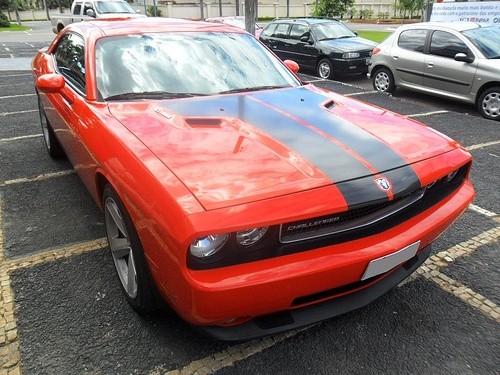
[50,1,146,33]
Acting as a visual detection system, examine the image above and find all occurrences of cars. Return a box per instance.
[32,16,474,343]
[205,17,262,42]
[259,17,379,79]
[370,22,500,121]
